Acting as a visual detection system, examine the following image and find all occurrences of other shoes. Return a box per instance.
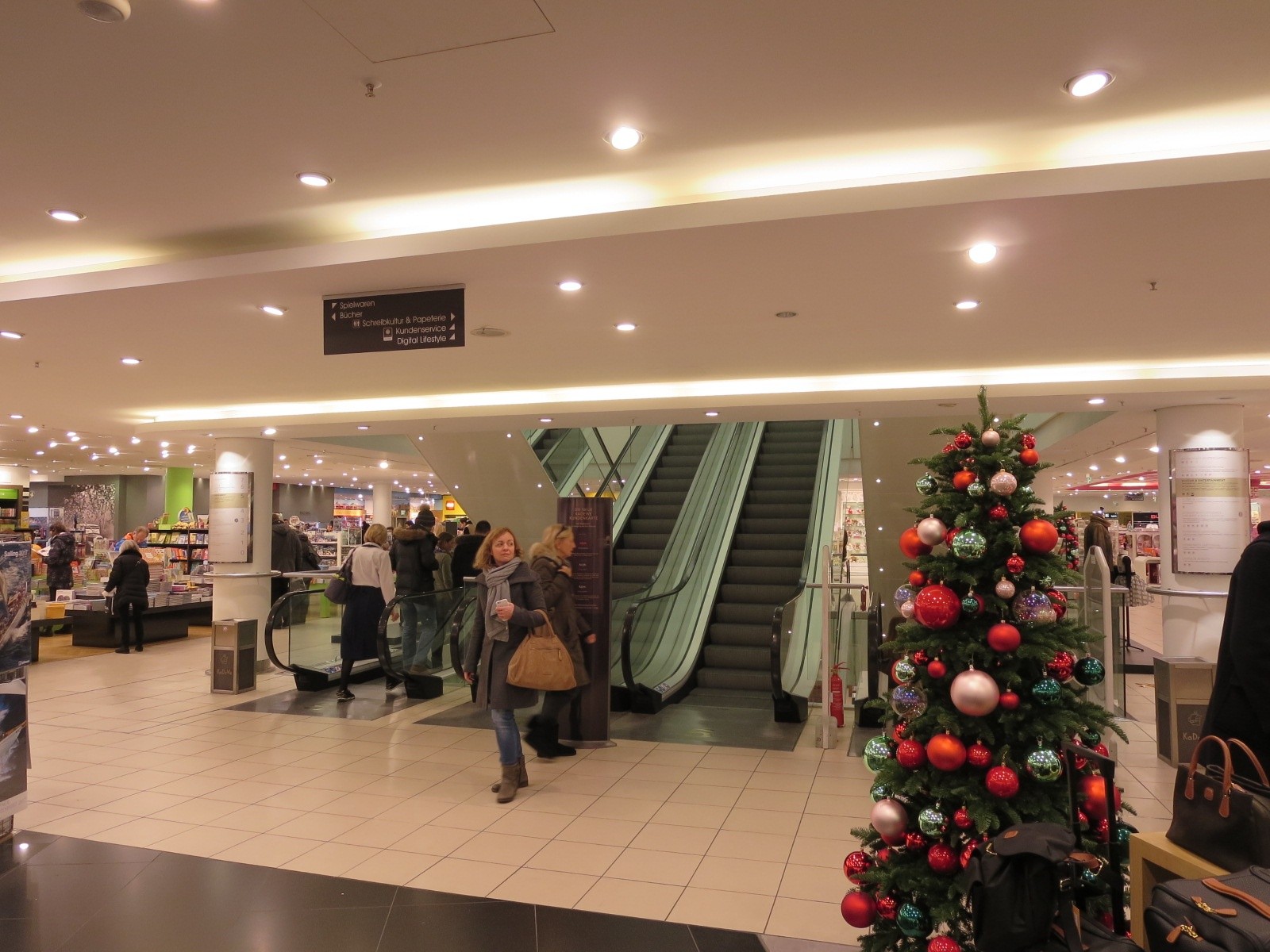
[115,646,130,653]
[136,645,143,652]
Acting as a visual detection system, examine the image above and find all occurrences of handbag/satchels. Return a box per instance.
[1164,735,1270,880]
[323,546,358,605]
[305,551,320,571]
[506,608,578,691]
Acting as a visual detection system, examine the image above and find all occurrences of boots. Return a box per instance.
[526,715,577,757]
[496,761,521,802]
[492,755,528,792]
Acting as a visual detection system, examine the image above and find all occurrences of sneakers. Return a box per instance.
[385,677,405,691]
[336,689,356,701]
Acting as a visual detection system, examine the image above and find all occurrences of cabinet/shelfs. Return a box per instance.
[30,616,75,662]
[305,529,363,571]
[145,529,209,575]
[66,601,213,649]
[842,501,868,565]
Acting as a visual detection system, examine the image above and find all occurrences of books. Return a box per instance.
[0,505,214,617]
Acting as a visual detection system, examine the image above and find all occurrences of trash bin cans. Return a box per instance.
[209,617,258,695]
[1151,653,1220,770]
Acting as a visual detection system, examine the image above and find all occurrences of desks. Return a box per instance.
[1135,556,1162,587]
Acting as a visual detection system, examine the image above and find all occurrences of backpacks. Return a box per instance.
[960,823,1102,952]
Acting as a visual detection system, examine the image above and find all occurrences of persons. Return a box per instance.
[335,521,403,703]
[460,525,551,803]
[1083,513,1115,584]
[1196,517,1269,785]
[104,539,149,654]
[432,532,454,669]
[1112,549,1155,608]
[263,509,474,627]
[387,503,441,672]
[450,519,493,588]
[521,523,598,758]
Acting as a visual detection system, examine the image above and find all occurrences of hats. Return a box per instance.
[272,513,279,523]
[416,503,436,526]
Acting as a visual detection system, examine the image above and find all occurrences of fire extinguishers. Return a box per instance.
[829,662,851,728]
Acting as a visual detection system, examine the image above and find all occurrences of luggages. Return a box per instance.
[1052,742,1144,951]
[1144,864,1269,952]
